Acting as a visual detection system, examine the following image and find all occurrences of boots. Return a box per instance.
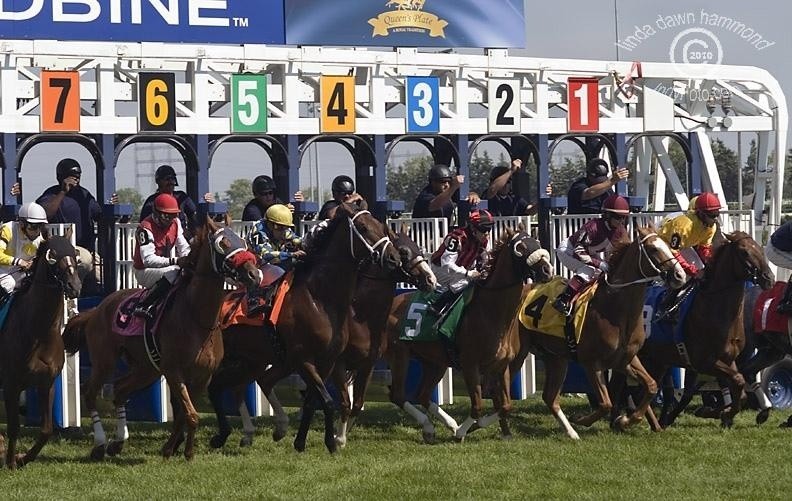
[246,287,272,318]
[429,289,455,314]
[552,275,584,313]
[654,283,687,324]
[777,280,792,314]
[135,276,171,318]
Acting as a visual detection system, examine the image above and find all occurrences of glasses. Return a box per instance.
[158,214,174,219]
[27,224,43,230]
[261,192,273,195]
[480,226,491,232]
[274,225,289,230]
[338,192,352,196]
[612,215,625,220]
[704,212,720,218]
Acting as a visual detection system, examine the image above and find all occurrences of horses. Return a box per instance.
[62,210,263,462]
[0,222,82,471]
[205,196,792,454]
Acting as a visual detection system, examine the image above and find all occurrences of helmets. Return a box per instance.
[696,193,721,211]
[56,159,81,179]
[586,158,608,177]
[19,202,49,224]
[155,165,176,184]
[490,167,509,182]
[253,176,276,191]
[469,209,493,233]
[263,204,295,226]
[333,176,354,192]
[430,164,453,183]
[601,195,630,216]
[154,194,181,213]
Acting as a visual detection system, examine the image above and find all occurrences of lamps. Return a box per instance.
[706,84,734,128]
